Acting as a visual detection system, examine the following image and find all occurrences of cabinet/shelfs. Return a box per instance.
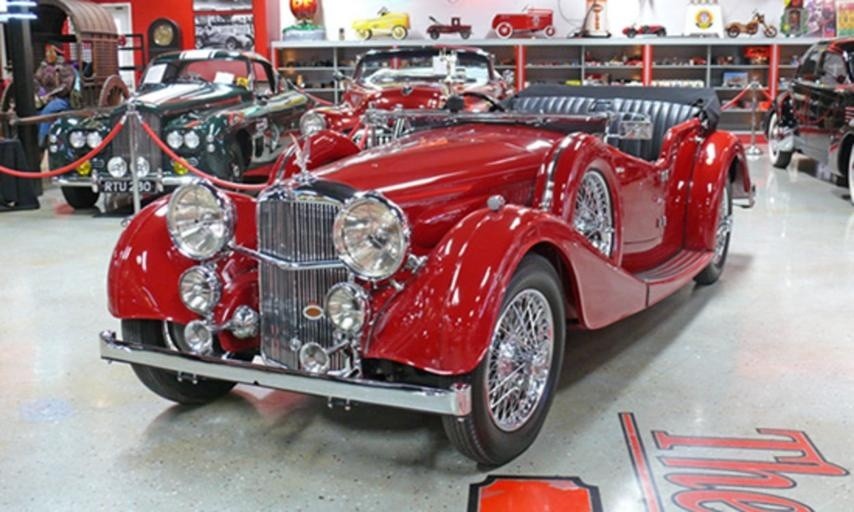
[271,39,813,148]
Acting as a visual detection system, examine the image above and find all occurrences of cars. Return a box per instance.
[196,26,254,49]
[766,37,854,207]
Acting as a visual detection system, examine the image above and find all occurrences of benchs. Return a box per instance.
[507,95,696,160]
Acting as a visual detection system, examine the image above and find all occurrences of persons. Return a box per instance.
[32,43,75,150]
